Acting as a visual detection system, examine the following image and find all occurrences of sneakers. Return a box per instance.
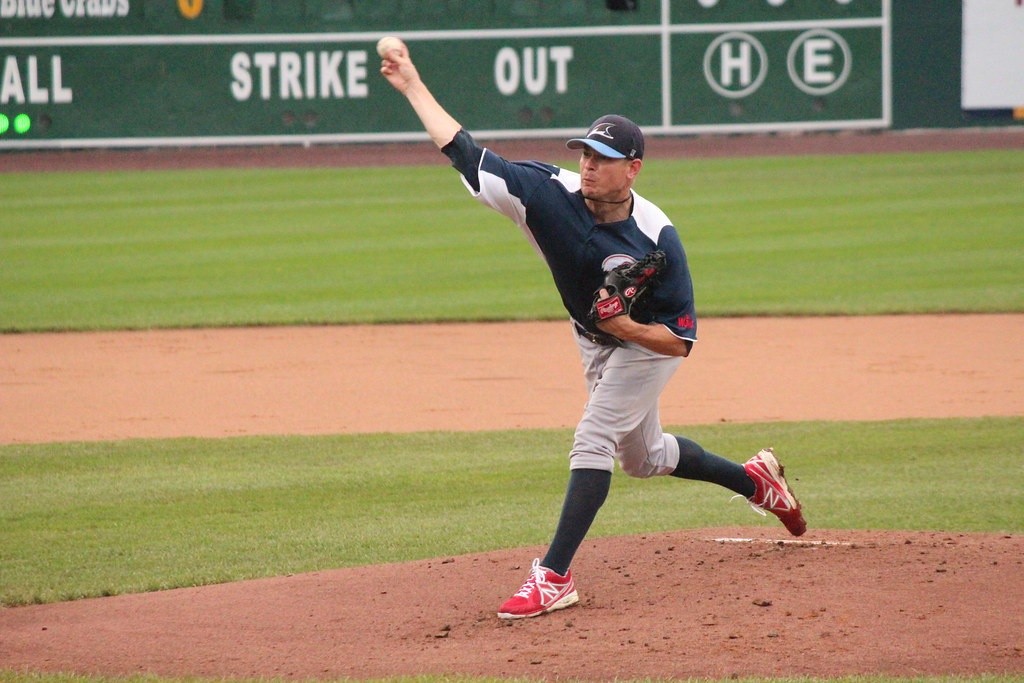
[496,557,580,619]
[729,447,808,537]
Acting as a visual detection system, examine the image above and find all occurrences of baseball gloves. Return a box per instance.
[582,247,668,325]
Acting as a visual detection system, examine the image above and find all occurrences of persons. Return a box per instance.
[379,40,808,618]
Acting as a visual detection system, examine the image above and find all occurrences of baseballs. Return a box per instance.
[376,34,406,62]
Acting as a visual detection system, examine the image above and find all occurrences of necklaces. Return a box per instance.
[572,188,633,204]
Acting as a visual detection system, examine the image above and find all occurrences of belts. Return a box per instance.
[572,317,629,348]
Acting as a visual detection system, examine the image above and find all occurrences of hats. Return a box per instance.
[566,114,646,162]
